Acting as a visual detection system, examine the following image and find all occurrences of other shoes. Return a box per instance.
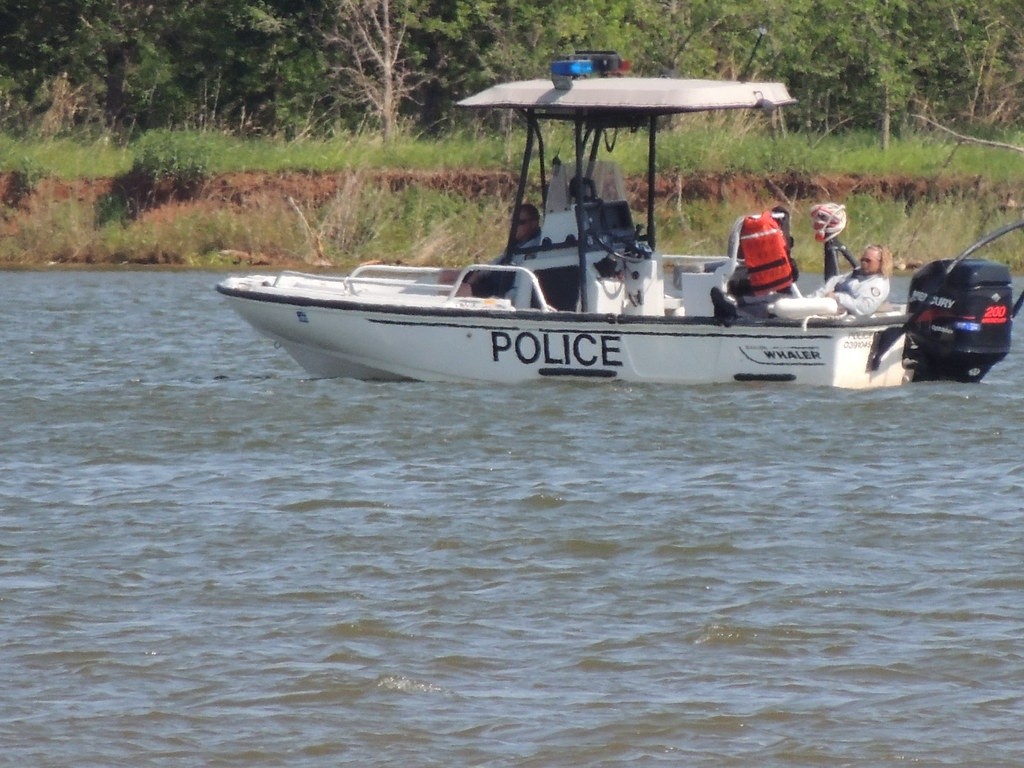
[709,286,736,317]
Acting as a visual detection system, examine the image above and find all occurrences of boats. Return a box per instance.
[215,46,1024,393]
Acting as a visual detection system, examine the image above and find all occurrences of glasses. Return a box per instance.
[517,218,535,225]
[860,257,872,263]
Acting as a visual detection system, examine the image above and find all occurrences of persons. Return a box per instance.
[710,244,893,327]
[455,205,541,298]
